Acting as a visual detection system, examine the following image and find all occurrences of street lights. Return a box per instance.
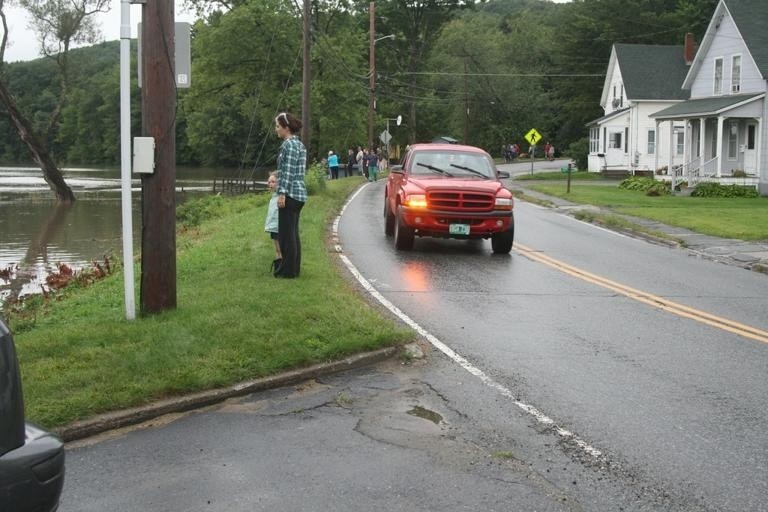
[366,34,399,176]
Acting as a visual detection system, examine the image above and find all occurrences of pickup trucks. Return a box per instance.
[381,142,519,255]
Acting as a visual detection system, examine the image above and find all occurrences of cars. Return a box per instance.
[1,319,69,512]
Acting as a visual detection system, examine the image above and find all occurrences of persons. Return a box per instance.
[320,145,388,183]
[272,110,310,280]
[500,139,557,164]
[262,169,281,270]
